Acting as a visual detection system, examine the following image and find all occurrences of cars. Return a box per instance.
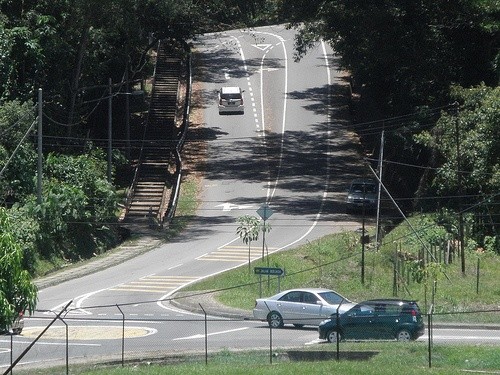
[319,298,426,344]
[253,287,375,329]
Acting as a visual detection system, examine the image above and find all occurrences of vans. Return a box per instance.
[347,178,378,212]
[216,86,245,114]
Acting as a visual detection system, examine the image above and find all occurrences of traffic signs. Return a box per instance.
[255,267,283,275]
